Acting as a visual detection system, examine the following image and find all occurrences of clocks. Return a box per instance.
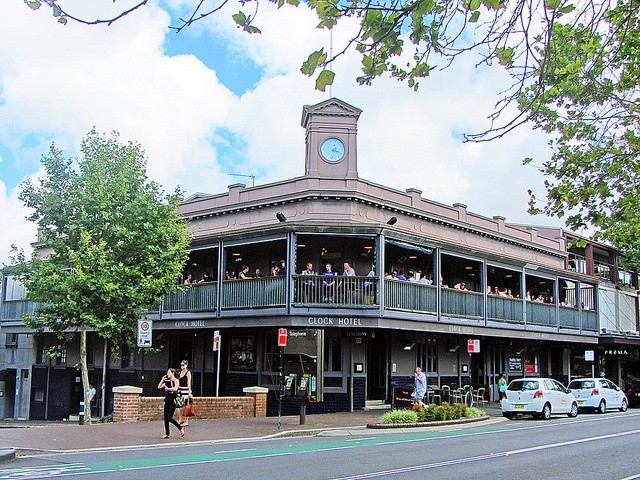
[321,138,345,161]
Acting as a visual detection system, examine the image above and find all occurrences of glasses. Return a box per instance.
[181,364,184,366]
[461,284,466,286]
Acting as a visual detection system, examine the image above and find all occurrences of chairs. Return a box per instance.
[424,384,485,408]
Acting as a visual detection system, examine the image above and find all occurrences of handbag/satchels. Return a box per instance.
[173,396,185,408]
[183,397,196,417]
[500,384,507,393]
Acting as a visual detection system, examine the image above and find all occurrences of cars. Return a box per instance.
[501,378,579,419]
[566,378,629,413]
[628,381,640,407]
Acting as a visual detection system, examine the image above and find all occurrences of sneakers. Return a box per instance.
[180,423,190,426]
[178,419,185,424]
[180,427,186,437]
[160,435,170,438]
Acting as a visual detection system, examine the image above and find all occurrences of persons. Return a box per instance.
[321,263,337,301]
[455,282,468,290]
[301,263,319,303]
[488,286,520,299]
[525,290,554,303]
[157,367,186,439]
[272,263,286,276]
[338,262,357,296]
[183,274,192,286]
[197,272,213,284]
[89,384,96,418]
[561,298,570,307]
[384,269,434,285]
[253,268,263,278]
[270,266,275,276]
[364,271,377,304]
[415,368,429,410]
[176,360,193,427]
[498,374,507,407]
[225,269,234,281]
[238,265,252,279]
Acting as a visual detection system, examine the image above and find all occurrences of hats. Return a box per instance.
[407,271,413,275]
